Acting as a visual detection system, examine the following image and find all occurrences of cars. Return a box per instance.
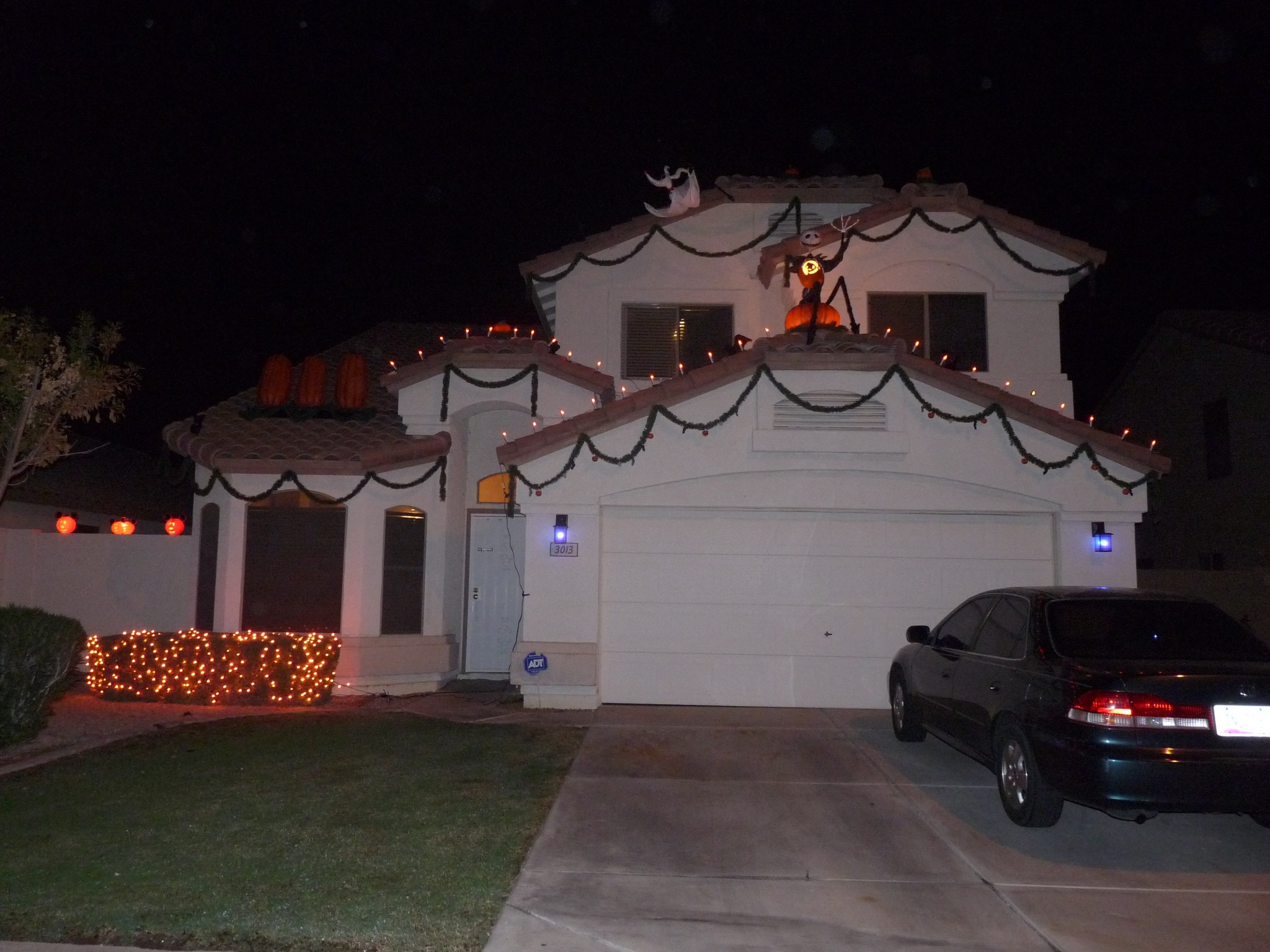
[885,585,1270,829]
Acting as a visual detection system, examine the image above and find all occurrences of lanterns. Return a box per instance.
[162,512,187,536]
[798,259,825,289]
[54,510,79,534]
[108,516,138,535]
